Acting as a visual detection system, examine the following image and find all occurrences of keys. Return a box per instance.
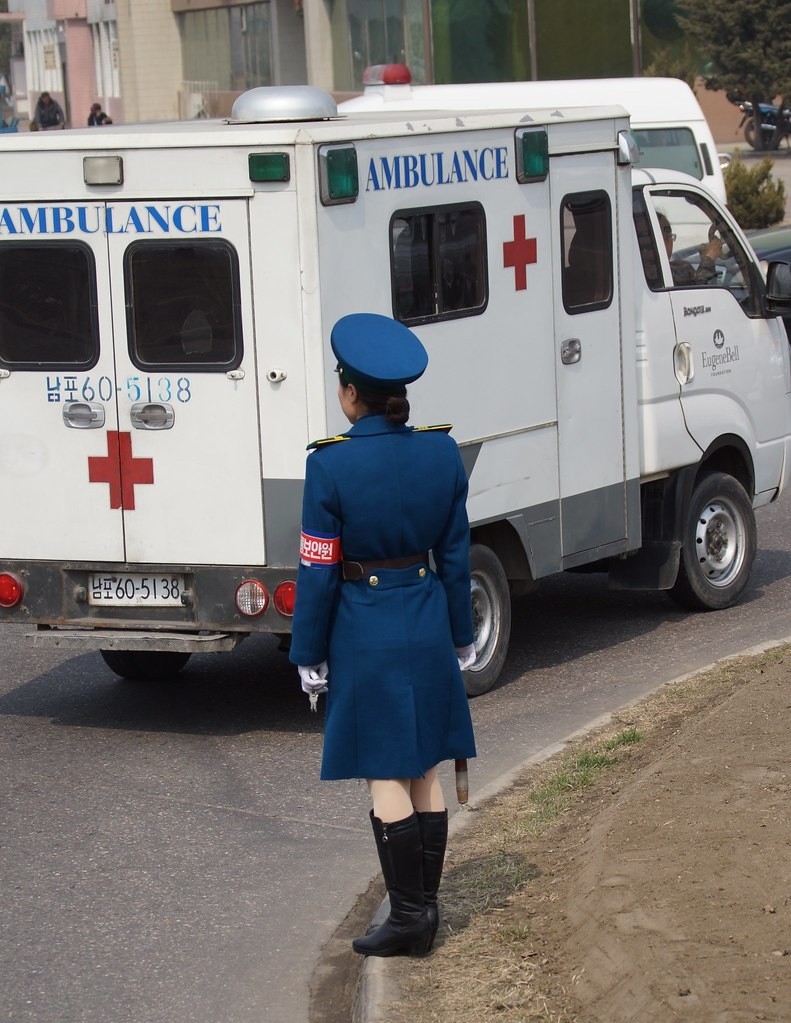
[309,691,318,712]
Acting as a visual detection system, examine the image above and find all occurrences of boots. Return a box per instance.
[352,808,448,958]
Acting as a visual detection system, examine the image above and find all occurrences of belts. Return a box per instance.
[339,554,429,580]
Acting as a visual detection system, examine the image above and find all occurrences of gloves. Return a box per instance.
[297,660,329,694]
[455,643,477,671]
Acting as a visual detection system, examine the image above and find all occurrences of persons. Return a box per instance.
[34,91,65,131]
[289,314,477,958]
[653,209,724,286]
[88,103,113,125]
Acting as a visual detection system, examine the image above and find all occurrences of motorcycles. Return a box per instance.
[735,99,791,149]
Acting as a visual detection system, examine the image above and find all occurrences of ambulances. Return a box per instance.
[0,83,791,698]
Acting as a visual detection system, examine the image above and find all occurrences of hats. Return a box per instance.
[330,312,428,392]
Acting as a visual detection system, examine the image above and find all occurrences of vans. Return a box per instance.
[335,61,732,267]
